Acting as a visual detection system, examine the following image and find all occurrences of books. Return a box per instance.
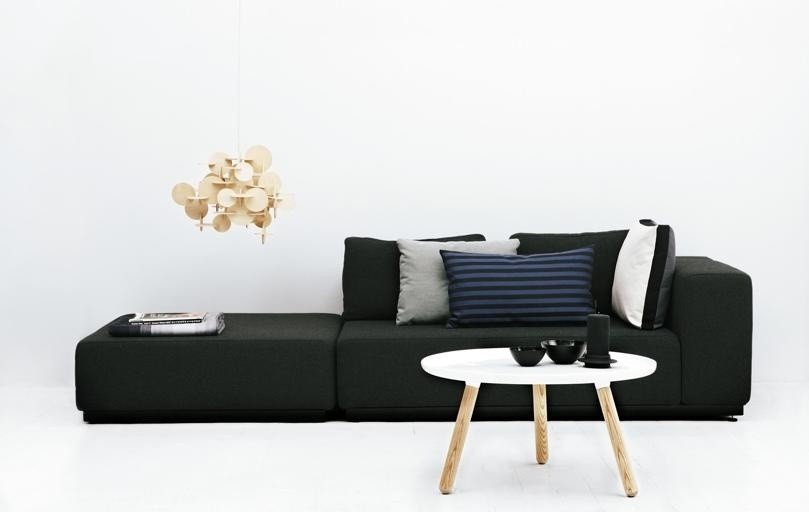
[127,308,209,326]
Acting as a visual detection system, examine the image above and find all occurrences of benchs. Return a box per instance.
[77,257,753,423]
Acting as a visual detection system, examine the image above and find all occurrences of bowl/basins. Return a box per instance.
[510,345,547,367]
[542,339,586,364]
